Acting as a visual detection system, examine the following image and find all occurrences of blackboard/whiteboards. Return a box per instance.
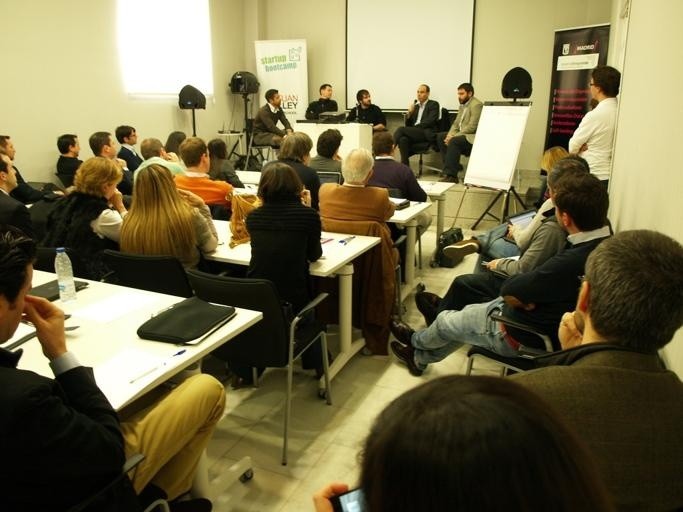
[463,102,533,191]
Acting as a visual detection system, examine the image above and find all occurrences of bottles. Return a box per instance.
[52,245,76,301]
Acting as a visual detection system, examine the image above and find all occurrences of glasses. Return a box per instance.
[577,275,584,281]
[588,82,596,87]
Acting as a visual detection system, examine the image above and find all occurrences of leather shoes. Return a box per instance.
[438,176,457,183]
[172,498,212,511]
[231,375,248,387]
[390,341,421,376]
[415,294,436,327]
[389,318,414,347]
[422,292,438,305]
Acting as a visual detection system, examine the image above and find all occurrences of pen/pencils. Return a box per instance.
[130,350,186,383]
[339,236,355,245]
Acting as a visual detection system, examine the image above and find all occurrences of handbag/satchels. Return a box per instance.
[430,227,463,268]
[229,194,262,248]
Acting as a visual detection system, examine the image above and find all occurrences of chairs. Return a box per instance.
[1,457,172,512]
[244,119,281,172]
[465,313,556,376]
[411,106,452,178]
[105,249,193,308]
[183,263,332,465]
[32,247,79,277]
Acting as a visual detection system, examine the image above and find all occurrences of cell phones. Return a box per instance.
[329,485,368,512]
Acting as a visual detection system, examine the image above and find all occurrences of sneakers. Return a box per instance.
[442,240,479,258]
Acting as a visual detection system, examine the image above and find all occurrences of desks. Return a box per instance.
[384,197,433,315]
[204,219,382,399]
[232,188,258,196]
[1,268,263,501]
[416,181,457,268]
[235,170,262,185]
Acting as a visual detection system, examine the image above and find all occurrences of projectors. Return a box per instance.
[318,111,346,122]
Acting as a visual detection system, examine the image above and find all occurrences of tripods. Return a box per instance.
[226,102,265,161]
[471,186,530,229]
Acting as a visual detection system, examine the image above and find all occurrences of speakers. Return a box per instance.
[501,66,533,98]
[179,85,206,109]
